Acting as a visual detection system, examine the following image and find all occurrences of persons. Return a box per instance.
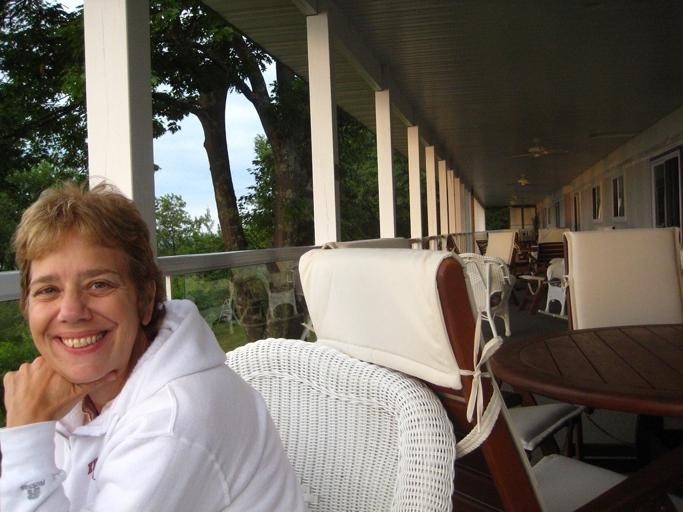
[0,178,306,511]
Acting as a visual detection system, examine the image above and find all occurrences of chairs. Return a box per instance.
[217,270,298,336]
[219,339,455,512]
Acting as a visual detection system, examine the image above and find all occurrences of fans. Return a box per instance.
[505,142,569,201]
